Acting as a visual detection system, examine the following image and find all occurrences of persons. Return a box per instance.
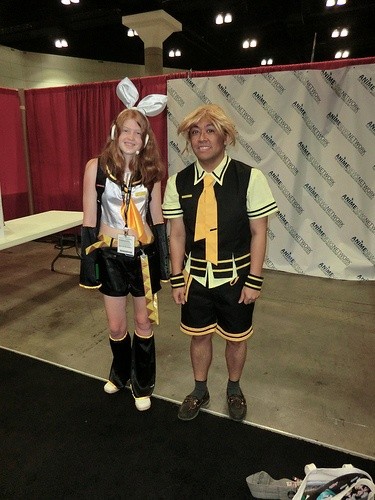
[78,77,171,411]
[162,104,277,421]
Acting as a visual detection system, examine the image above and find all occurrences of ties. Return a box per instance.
[193,174,217,266]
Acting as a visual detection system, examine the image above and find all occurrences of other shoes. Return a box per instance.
[130,384,151,410]
[226,387,247,421]
[104,380,120,394]
[178,387,209,420]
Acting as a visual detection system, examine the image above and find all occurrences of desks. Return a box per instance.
[0,210,84,271]
